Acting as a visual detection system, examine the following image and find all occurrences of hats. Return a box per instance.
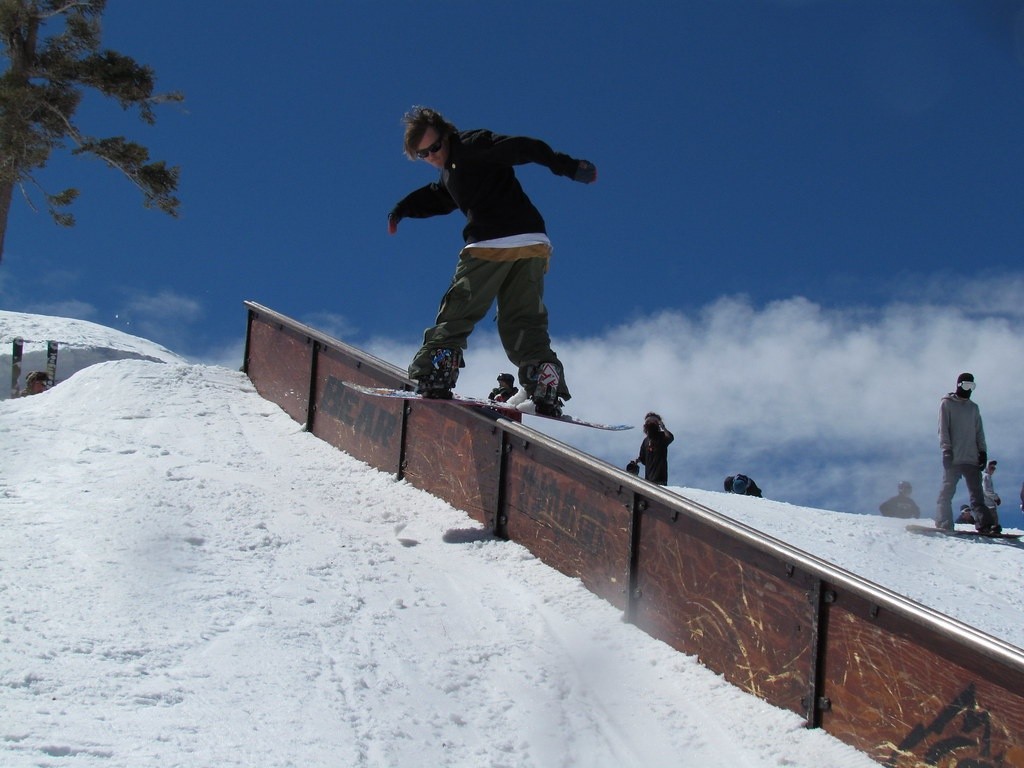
[959,504,970,514]
[733,480,746,493]
[497,372,514,388]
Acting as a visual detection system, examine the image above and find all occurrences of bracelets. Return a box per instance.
[662,425,665,429]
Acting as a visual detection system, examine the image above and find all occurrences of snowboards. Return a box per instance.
[340,379,635,432]
[906,525,1024,538]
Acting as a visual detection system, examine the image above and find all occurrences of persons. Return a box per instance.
[488,373,522,424]
[935,373,1002,532]
[724,474,763,498]
[981,460,1001,524]
[955,504,975,525]
[626,413,674,486]
[21,371,47,397]
[879,481,920,519]
[388,110,596,416]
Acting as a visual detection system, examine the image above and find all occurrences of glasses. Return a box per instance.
[958,381,976,391]
[989,464,996,470]
[499,373,503,377]
[415,135,443,158]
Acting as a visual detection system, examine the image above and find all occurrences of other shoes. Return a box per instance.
[935,520,954,531]
[414,347,455,399]
[531,361,565,418]
[978,524,1002,534]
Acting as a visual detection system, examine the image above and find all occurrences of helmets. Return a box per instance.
[898,481,911,494]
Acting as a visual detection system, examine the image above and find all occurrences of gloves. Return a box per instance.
[979,451,987,471]
[995,497,1001,505]
[942,449,953,469]
